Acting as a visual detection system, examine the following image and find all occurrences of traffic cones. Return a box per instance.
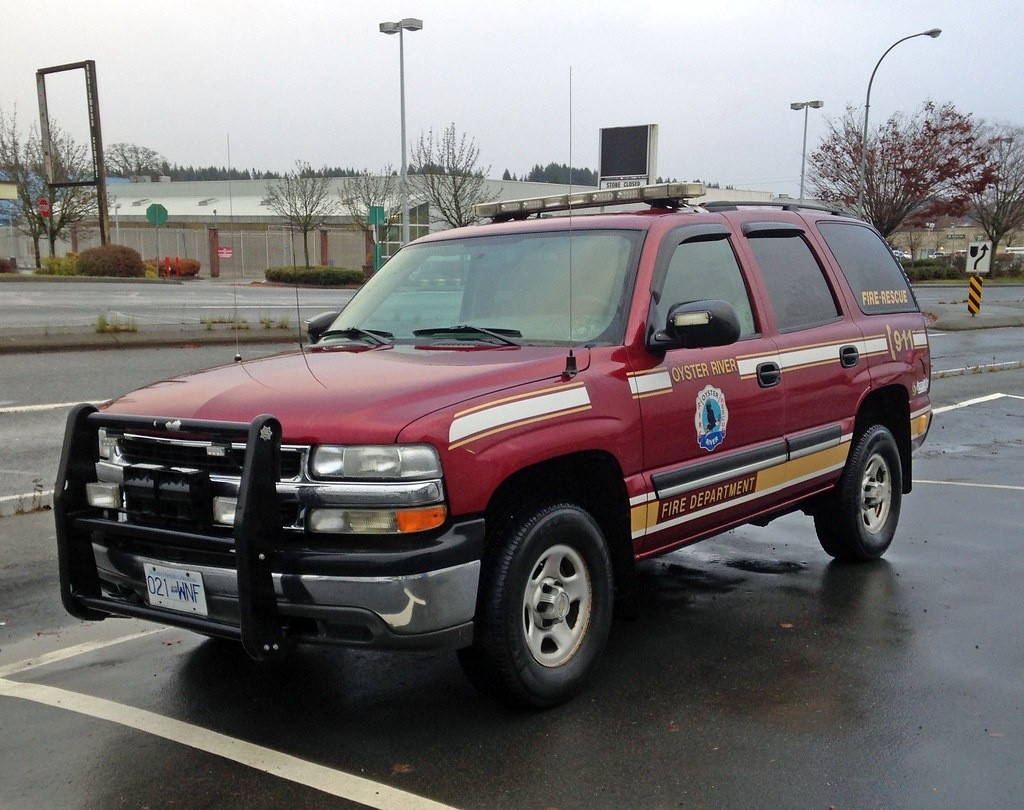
[164,256,173,277]
[175,256,183,277]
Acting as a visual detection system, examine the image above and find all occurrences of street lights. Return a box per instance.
[115,203,121,244]
[379,18,423,245]
[791,101,824,205]
[858,28,941,218]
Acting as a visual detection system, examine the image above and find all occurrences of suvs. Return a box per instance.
[52,181,932,709]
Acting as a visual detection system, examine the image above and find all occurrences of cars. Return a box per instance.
[892,251,911,260]
[955,250,966,256]
[928,252,951,258]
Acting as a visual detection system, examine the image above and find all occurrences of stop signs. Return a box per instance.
[40,199,50,218]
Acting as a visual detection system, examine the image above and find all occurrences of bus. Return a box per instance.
[1005,247,1024,254]
